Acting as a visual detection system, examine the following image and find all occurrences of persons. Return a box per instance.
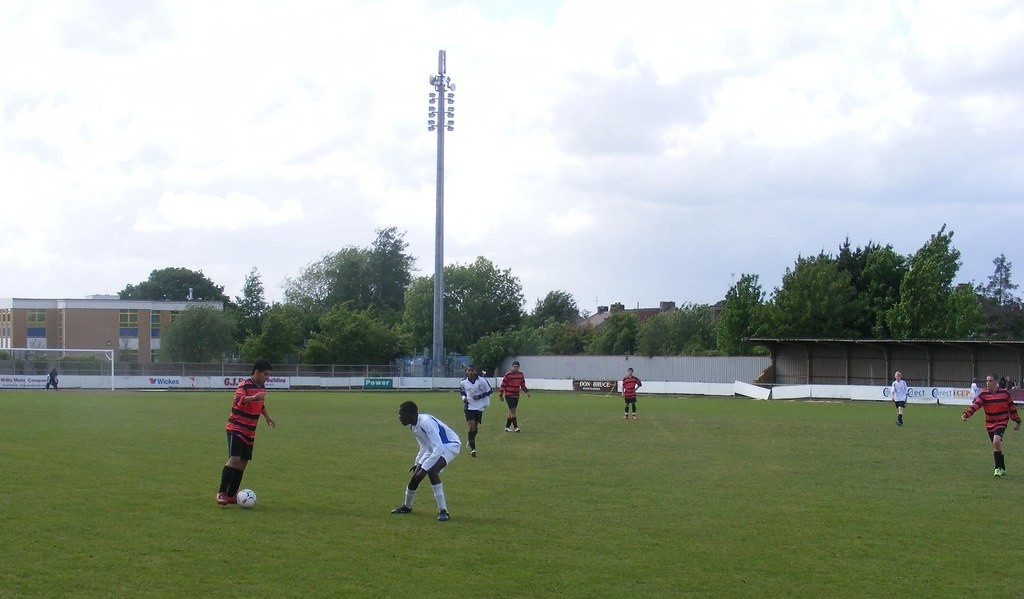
[389,400,461,521]
[422,354,430,376]
[459,364,493,457]
[890,372,908,426]
[498,361,530,432]
[445,352,454,377]
[621,367,642,420]
[44,367,59,392]
[961,373,1022,477]
[217,359,276,505]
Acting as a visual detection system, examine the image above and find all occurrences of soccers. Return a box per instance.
[236,488,258,510]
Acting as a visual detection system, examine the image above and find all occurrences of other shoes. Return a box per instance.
[895,420,902,427]
[632,415,636,420]
[623,415,628,419]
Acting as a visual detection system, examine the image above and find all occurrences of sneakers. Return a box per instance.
[470,448,477,457]
[391,504,412,514]
[993,467,1001,476]
[1000,468,1008,476]
[226,493,237,503]
[514,427,521,433]
[436,509,450,520]
[466,440,471,448]
[504,427,513,432]
[216,491,228,505]
